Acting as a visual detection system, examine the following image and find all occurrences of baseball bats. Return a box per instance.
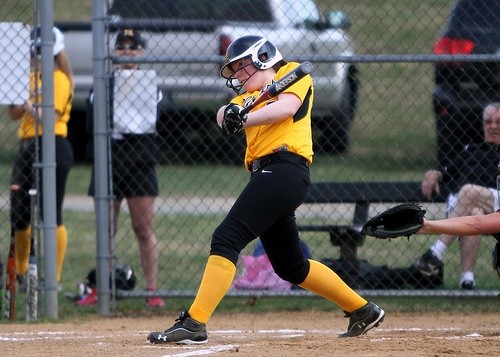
[25,188,38,322]
[238,62,314,114]
[5,185,20,320]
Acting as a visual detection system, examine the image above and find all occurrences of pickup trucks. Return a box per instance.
[54,0,360,165]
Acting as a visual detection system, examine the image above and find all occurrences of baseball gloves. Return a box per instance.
[362,201,426,240]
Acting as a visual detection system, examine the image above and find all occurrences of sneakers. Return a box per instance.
[75,288,97,306]
[147,310,208,344]
[338,302,384,337]
[146,287,164,308]
[461,280,475,289]
[412,251,440,275]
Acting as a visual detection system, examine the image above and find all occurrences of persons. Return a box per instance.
[148,35,386,345]
[7,25,74,291]
[361,201,500,242]
[416,103,500,289]
[75,27,165,308]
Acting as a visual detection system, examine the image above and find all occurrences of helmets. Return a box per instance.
[31,24,64,59]
[220,36,282,78]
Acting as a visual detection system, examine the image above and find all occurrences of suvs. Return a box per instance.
[433,0,500,155]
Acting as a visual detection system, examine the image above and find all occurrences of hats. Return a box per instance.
[115,30,145,48]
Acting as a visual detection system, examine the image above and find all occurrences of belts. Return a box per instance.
[249,150,310,175]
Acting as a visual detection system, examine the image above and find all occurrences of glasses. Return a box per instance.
[115,44,141,50]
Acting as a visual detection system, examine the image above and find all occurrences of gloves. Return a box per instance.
[221,121,243,137]
[224,105,248,123]
